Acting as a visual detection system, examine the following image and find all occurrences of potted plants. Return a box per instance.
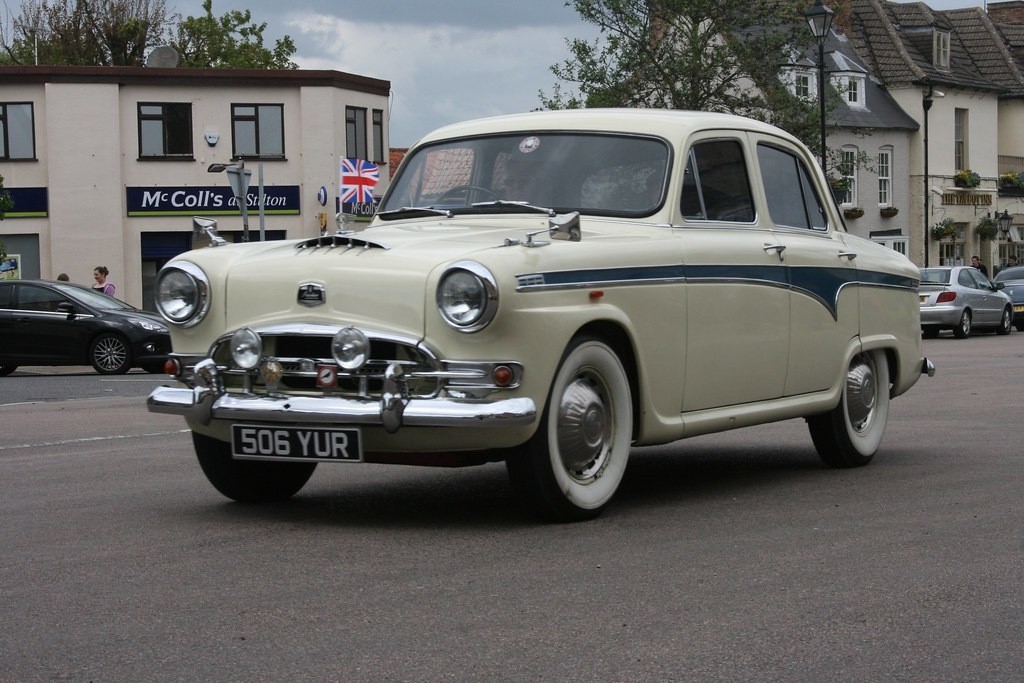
[844,207,864,219]
[880,206,898,217]
[830,178,849,201]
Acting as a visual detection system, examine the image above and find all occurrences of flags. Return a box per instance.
[341,159,380,203]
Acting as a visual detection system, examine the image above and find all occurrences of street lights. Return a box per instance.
[207,160,249,243]
[805,0,837,172]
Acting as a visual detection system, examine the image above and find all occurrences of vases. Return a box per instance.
[956,179,976,187]
[1001,183,1019,188]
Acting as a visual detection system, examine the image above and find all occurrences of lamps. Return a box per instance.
[206,132,219,146]
[995,207,1013,234]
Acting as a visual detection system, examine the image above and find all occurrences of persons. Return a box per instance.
[1004,255,1020,268]
[57,273,69,282]
[970,256,989,278]
[92,266,115,297]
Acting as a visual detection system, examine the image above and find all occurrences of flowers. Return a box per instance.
[998,170,1024,184]
[934,222,955,239]
[953,169,981,188]
[977,220,998,237]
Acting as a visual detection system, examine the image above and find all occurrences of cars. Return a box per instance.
[0,279,173,376]
[917,266,1015,339]
[991,266,1024,333]
[147,107,935,524]
